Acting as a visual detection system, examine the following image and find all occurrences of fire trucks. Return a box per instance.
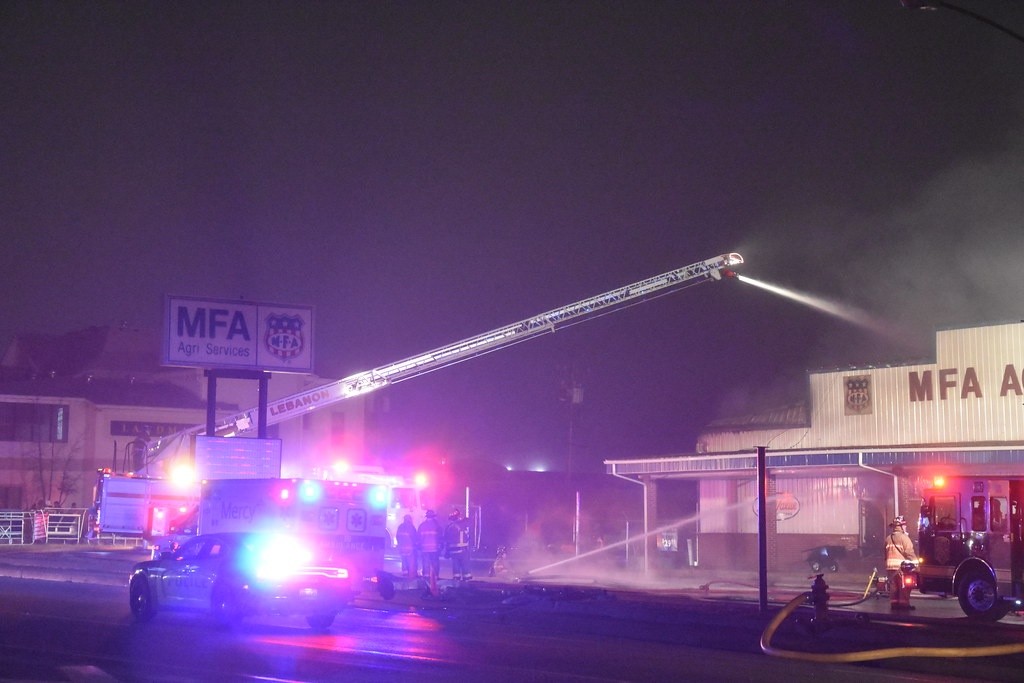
[90,251,745,544]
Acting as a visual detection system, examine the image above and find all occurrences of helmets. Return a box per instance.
[404,515,411,520]
[425,510,436,517]
[448,509,460,519]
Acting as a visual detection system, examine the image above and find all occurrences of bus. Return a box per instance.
[917,477,1024,621]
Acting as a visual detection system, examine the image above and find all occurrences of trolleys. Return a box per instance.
[798,544,845,572]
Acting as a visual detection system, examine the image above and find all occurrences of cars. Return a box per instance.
[128,530,353,629]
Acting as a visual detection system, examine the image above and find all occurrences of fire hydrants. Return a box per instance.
[808,574,833,636]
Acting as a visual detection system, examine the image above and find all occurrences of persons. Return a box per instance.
[444,509,473,583]
[494,548,517,579]
[418,510,443,580]
[885,515,917,612]
[29,498,80,514]
[395,515,418,578]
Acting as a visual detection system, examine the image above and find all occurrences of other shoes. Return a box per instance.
[890,601,899,609]
[899,603,916,610]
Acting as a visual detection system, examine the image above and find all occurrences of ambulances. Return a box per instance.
[150,479,388,583]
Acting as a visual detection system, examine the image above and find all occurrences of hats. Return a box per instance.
[889,515,906,526]
